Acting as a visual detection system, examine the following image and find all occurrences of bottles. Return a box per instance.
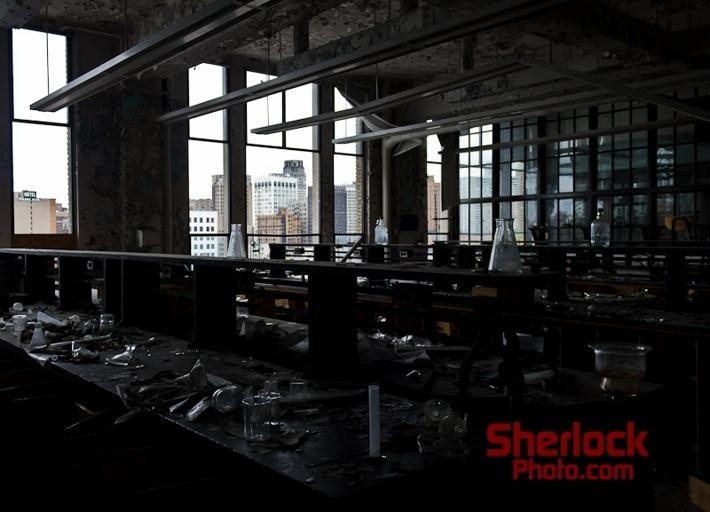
[486,217,524,273]
[373,217,390,245]
[29,322,48,349]
[367,386,381,458]
[589,208,613,248]
[235,295,249,319]
[225,222,247,260]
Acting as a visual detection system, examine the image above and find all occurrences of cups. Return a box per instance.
[99,313,116,332]
[12,315,28,337]
[211,371,309,443]
[268,449,294,476]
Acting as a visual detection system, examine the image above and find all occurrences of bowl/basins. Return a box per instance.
[588,340,657,379]
